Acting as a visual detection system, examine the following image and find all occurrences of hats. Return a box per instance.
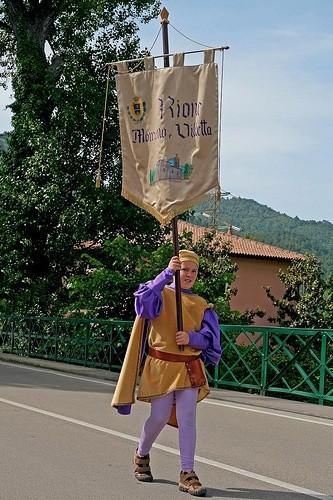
[179,250,199,267]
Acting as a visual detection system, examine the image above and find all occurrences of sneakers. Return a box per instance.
[179,470,207,496]
[132,447,153,482]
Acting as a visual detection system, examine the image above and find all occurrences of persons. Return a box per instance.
[110,248,222,497]
